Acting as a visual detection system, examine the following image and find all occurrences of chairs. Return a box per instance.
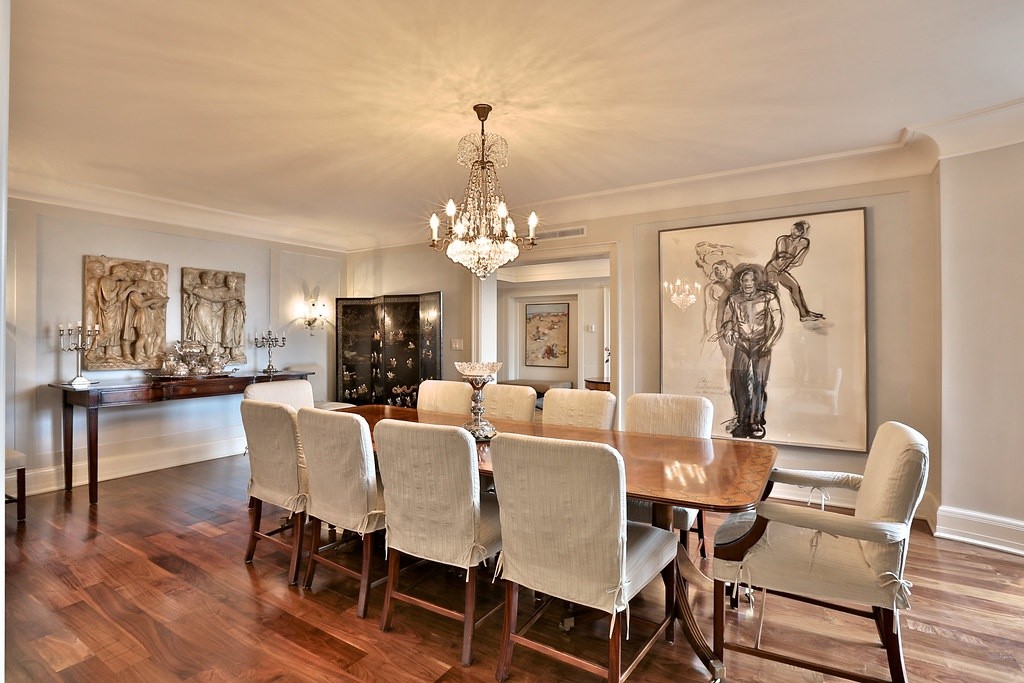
[767,421,930,683]
[481,383,537,423]
[542,388,616,429]
[417,380,473,416]
[627,391,713,597]
[244,379,315,412]
[240,399,309,585]
[491,431,677,683]
[296,405,386,618]
[373,418,501,665]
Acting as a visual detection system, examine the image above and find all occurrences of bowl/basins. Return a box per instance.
[454,362,504,376]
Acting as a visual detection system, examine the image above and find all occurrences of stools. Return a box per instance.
[5,448,27,520]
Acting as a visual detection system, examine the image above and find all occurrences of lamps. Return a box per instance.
[59,320,100,385]
[430,105,538,281]
[255,325,286,373]
[300,299,326,335]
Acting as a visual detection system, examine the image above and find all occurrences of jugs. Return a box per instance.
[160,339,231,377]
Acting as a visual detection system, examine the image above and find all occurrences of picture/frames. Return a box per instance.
[525,303,570,368]
[657,208,869,453]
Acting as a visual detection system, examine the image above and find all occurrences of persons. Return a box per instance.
[184,271,246,360]
[88,260,170,365]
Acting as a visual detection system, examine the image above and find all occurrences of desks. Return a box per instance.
[497,379,571,410]
[583,377,610,391]
[48,368,315,503]
[332,404,779,644]
